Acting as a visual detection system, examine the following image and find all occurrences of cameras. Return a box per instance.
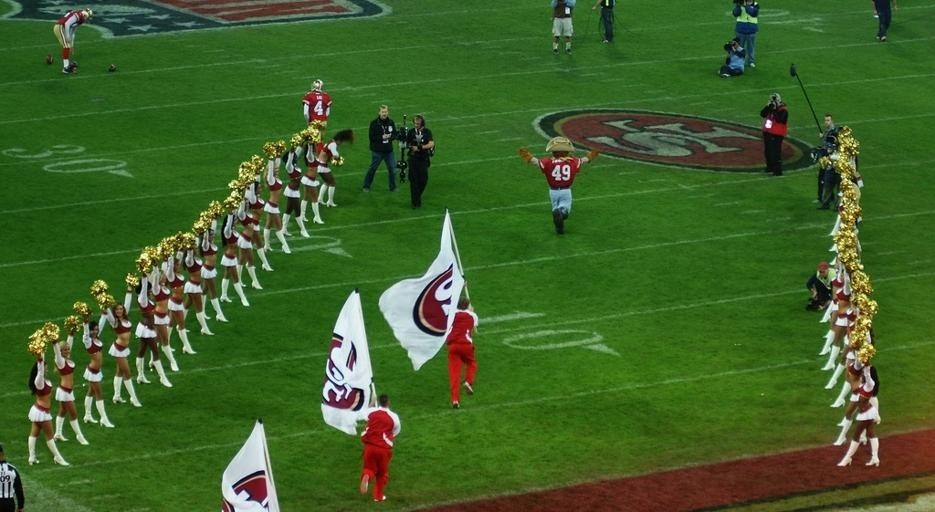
[726,41,736,46]
[767,94,777,109]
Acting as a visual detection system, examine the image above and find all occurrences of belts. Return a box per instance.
[550,186,568,191]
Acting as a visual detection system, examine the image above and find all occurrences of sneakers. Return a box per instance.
[63,65,75,73]
[462,381,473,395]
[452,401,460,408]
[360,475,369,495]
[374,496,387,502]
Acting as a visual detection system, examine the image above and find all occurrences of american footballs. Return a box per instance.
[46,54,53,65]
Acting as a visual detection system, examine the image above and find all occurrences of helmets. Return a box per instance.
[312,79,325,91]
[82,8,94,22]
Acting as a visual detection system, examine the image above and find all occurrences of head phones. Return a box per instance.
[412,114,425,127]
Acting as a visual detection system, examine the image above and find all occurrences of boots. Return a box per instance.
[812,179,880,467]
[552,207,569,235]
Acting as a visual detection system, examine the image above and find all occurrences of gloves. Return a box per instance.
[587,149,600,164]
[519,148,533,163]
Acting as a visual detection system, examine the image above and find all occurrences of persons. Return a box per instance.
[404,113,435,211]
[442,295,487,409]
[871,1,899,43]
[301,78,333,127]
[519,138,601,237]
[363,103,401,194]
[0,445,28,510]
[361,392,404,504]
[590,0,616,45]
[732,0,760,68]
[757,92,791,175]
[550,0,579,55]
[27,121,353,474]
[806,113,881,469]
[52,6,98,75]
[717,36,746,79]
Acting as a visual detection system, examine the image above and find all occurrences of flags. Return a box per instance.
[220,418,283,511]
[317,286,379,435]
[378,209,466,371]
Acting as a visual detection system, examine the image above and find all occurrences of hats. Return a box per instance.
[545,136,576,153]
